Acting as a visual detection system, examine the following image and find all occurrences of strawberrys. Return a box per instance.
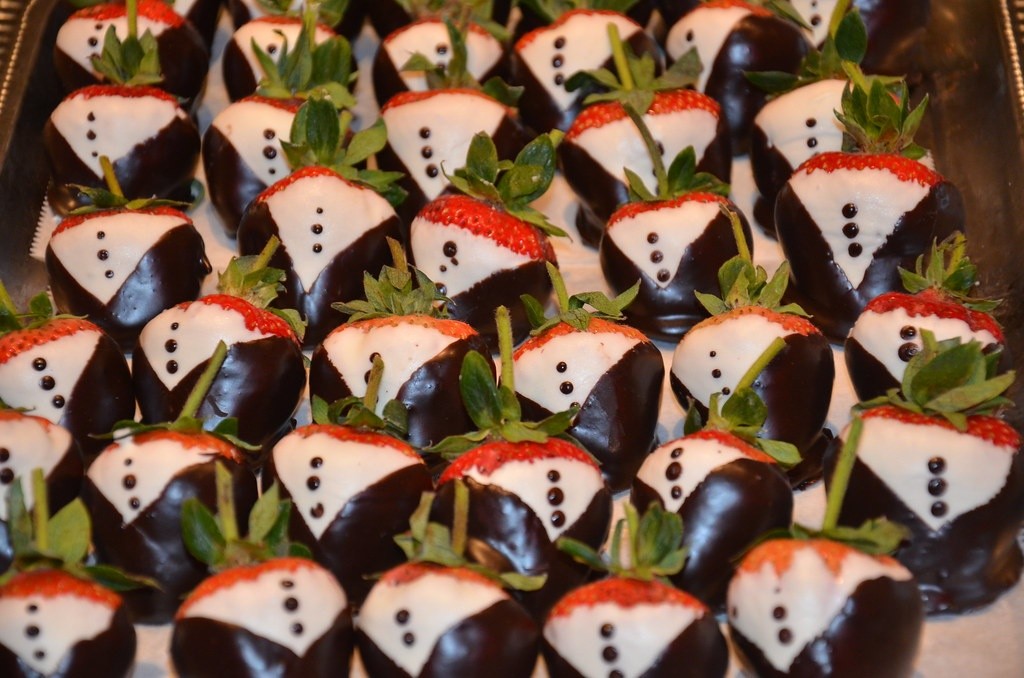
[0,0,1024,678]
